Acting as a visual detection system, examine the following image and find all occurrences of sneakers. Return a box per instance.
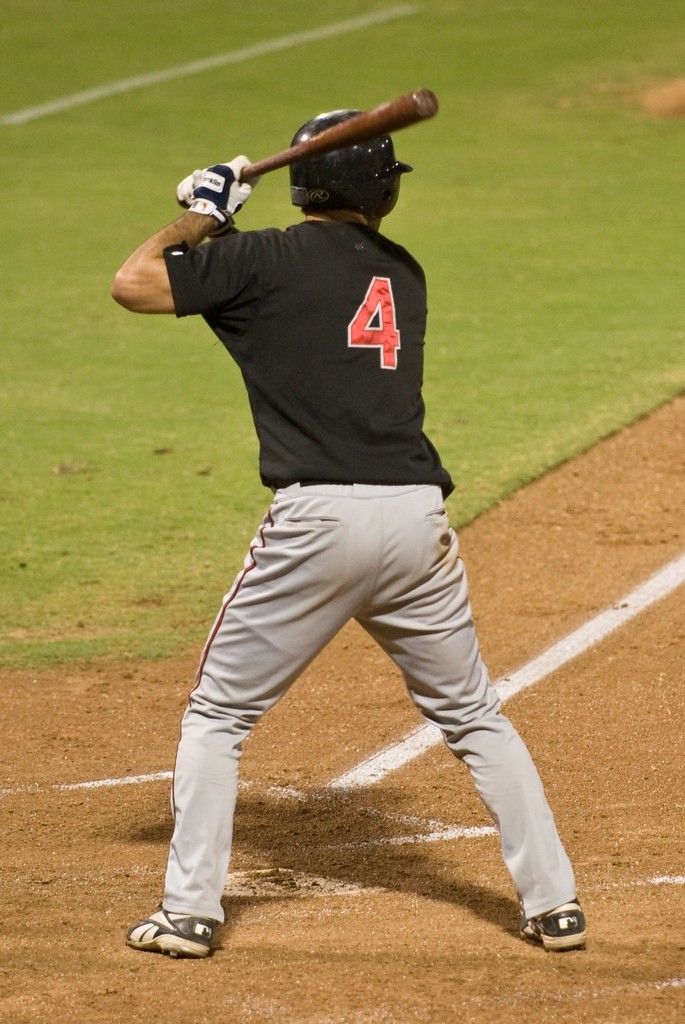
[521,898,588,951]
[127,907,218,959]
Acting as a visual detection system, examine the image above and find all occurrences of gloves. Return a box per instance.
[175,151,256,224]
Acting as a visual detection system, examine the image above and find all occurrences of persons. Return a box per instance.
[111,109,589,961]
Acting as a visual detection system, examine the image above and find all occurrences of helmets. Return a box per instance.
[288,108,418,218]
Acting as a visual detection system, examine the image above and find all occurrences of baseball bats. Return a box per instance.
[176,87,438,209]
